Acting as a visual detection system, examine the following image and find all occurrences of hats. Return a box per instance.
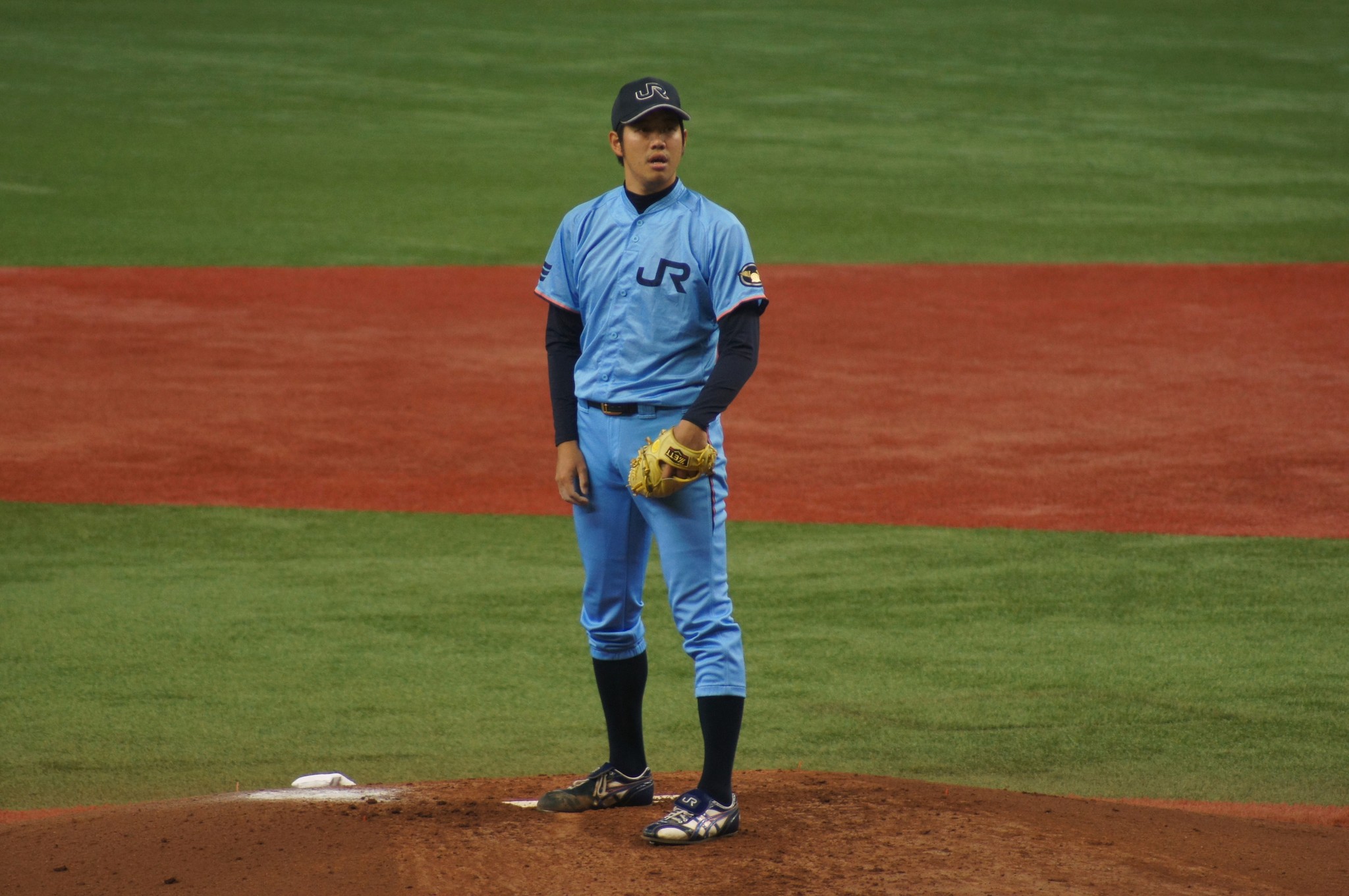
[612,77,690,131]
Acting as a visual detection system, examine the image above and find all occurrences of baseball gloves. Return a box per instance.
[629,427,718,498]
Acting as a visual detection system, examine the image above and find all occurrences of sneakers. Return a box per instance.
[536,761,653,811]
[642,788,741,844]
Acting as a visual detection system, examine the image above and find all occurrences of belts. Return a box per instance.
[587,401,674,415]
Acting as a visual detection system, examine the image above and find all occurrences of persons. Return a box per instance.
[537,75,769,845]
